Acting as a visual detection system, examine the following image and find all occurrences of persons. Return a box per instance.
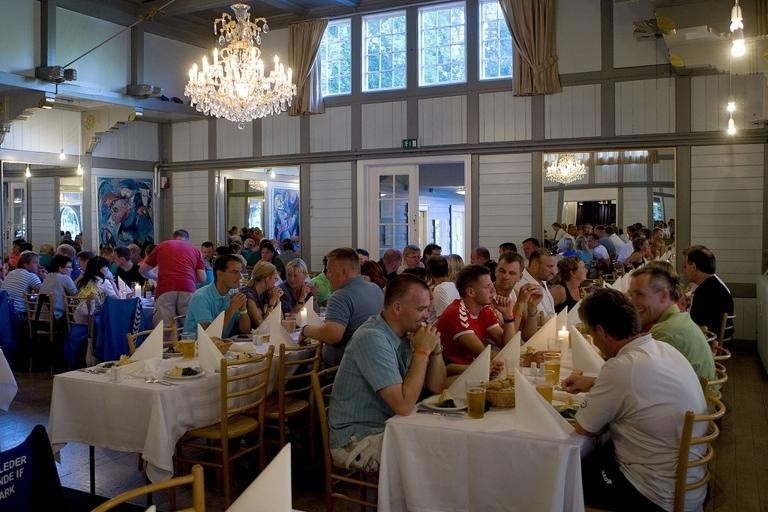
[625,269,719,414]
[224,225,301,263]
[685,248,735,352]
[683,246,732,294]
[561,287,710,511]
[0,230,207,342]
[545,217,672,270]
[208,225,587,471]
[646,261,686,311]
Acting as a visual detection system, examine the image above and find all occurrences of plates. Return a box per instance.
[96,360,139,370]
[165,369,206,379]
[424,394,469,412]
[163,352,182,358]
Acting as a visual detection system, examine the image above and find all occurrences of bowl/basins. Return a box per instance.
[485,378,515,407]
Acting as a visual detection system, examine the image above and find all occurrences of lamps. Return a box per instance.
[732,30,745,57]
[730,0,744,32]
[727,34,737,112]
[59,110,66,161]
[25,164,32,178]
[91,167,154,256]
[75,124,83,176]
[726,112,737,135]
[544,152,588,186]
[183,1,298,131]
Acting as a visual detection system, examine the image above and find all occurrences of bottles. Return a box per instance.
[144,278,151,300]
[131,282,136,294]
[151,282,155,297]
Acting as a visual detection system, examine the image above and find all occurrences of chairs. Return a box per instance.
[702,325,717,343]
[169,345,275,512]
[126,324,178,470]
[62,292,95,364]
[676,378,725,512]
[169,315,186,343]
[310,366,377,512]
[711,340,732,368]
[701,364,728,418]
[244,337,323,476]
[720,313,738,346]
[1,425,149,511]
[22,290,55,378]
[90,464,205,512]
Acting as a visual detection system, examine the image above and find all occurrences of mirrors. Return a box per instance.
[209,164,301,267]
[539,146,677,285]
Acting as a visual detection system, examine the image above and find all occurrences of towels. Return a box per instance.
[269,312,290,350]
[491,331,521,377]
[118,276,130,297]
[522,313,557,351]
[197,322,225,371]
[570,325,606,375]
[612,263,644,292]
[129,321,164,359]
[568,299,583,326]
[448,344,491,403]
[224,442,293,512]
[556,305,568,330]
[306,310,323,328]
[195,311,225,341]
[294,296,315,327]
[514,367,576,440]
[258,302,282,341]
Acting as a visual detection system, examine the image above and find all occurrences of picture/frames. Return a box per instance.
[268,182,300,252]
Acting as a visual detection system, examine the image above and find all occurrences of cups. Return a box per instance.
[466,381,486,419]
[544,354,560,386]
[535,374,552,405]
[180,307,327,361]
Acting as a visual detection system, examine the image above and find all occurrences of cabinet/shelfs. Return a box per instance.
[732,298,756,341]
[756,277,767,370]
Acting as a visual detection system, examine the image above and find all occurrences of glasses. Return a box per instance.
[406,255,421,258]
[63,266,72,268]
[271,277,279,282]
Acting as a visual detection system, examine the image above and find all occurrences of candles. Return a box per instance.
[299,306,308,325]
[135,283,141,297]
[559,326,570,351]
